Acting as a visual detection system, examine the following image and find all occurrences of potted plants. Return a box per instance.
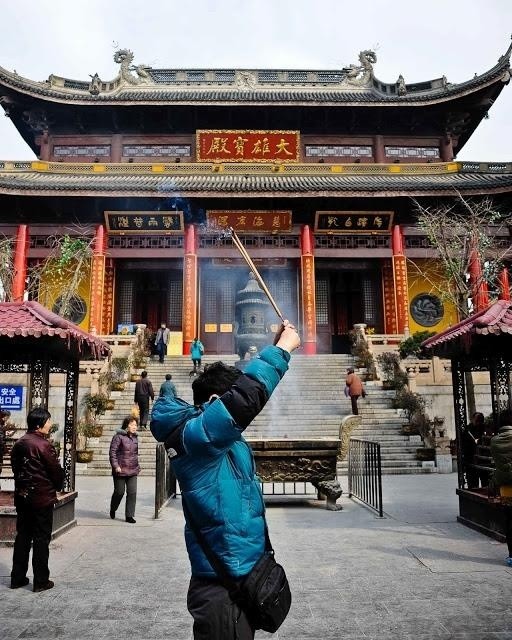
[74,330,153,464]
[347,330,437,467]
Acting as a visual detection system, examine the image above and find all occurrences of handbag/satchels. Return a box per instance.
[235,554,291,633]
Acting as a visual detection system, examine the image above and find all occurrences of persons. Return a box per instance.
[153,322,170,362]
[190,335,206,370]
[12,407,66,592]
[491,410,511,565]
[160,374,177,398]
[148,320,302,640]
[344,368,366,415]
[1,411,10,472]
[463,413,489,489]
[134,371,154,428]
[110,416,140,523]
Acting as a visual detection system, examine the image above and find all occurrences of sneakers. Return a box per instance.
[110,510,115,519]
[126,517,136,523]
[11,578,29,589]
[33,580,54,592]
